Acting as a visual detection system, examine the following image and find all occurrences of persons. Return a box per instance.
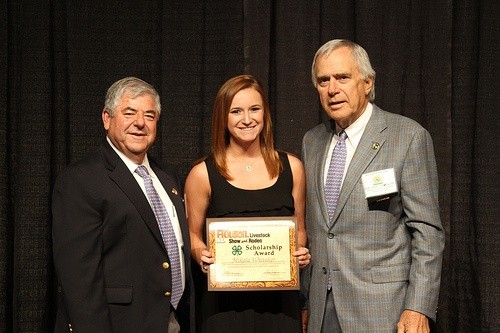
[183,74,312,333]
[52,77,194,333]
[301,38,445,333]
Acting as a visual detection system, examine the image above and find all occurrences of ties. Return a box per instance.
[133,164,184,311]
[323,131,348,291]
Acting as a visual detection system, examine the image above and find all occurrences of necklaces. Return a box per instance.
[232,155,266,177]
